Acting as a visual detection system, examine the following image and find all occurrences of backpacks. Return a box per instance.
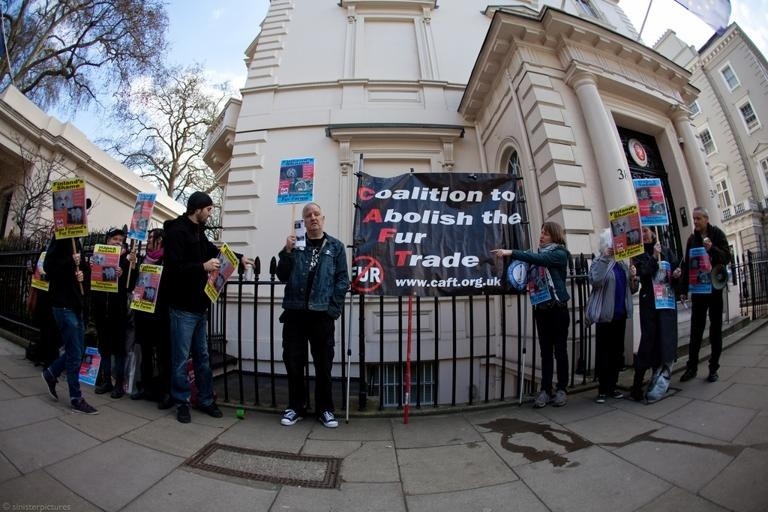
[642,361,673,405]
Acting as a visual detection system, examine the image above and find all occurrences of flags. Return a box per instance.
[673,0,731,37]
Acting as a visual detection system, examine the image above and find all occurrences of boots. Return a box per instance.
[680,360,697,382]
[707,364,720,383]
[94,375,114,394]
[111,375,125,398]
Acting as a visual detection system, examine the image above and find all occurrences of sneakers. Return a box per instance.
[70,397,99,415]
[609,390,623,398]
[534,390,549,407]
[555,390,566,405]
[177,405,191,423]
[320,411,338,428]
[281,409,304,426]
[41,368,60,401]
[199,401,223,418]
[158,392,175,409]
[596,395,607,402]
[131,390,144,400]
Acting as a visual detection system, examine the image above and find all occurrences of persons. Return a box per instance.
[38,199,175,414]
[629,226,686,402]
[588,229,643,404]
[163,191,256,424]
[489,220,572,407]
[675,206,732,383]
[275,202,349,428]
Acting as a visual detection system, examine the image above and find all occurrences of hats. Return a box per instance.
[187,192,213,213]
[109,227,122,233]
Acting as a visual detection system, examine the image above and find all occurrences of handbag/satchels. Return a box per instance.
[585,280,604,325]
